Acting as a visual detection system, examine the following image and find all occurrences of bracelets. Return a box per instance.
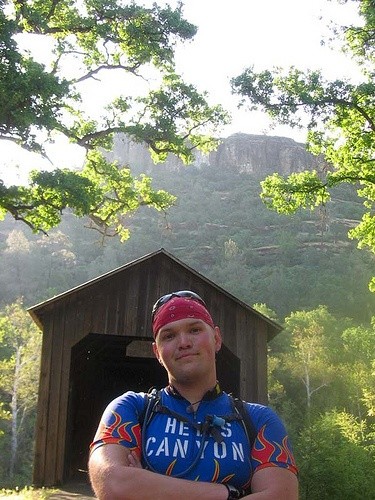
[224,484,241,500]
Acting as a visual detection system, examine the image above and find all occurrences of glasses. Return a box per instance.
[150,290,207,321]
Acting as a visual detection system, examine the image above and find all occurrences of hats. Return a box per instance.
[152,292,215,339]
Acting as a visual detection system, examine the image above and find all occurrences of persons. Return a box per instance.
[88,290,298,500]
